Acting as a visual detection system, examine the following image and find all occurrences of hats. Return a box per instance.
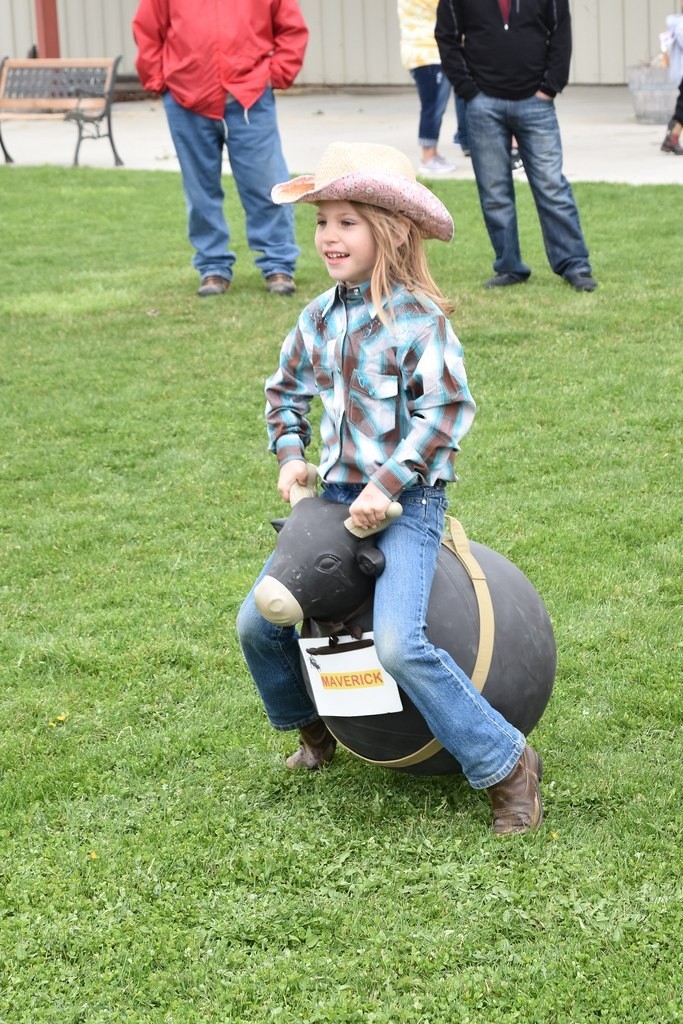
[270,140,454,243]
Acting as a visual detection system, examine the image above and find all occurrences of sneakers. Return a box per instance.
[197,275,229,296]
[484,273,518,289]
[267,273,296,295]
[420,154,455,174]
[568,272,597,291]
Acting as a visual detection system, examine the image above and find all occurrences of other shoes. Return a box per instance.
[510,147,525,173]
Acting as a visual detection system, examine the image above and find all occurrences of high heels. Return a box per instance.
[660,134,683,155]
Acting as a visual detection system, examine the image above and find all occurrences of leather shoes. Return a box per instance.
[486,746,543,837]
[285,727,337,772]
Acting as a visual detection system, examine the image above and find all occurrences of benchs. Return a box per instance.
[0,56,123,166]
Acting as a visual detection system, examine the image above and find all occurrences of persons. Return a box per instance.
[397,0,597,294]
[133,1,309,295]
[660,0,683,156]
[236,140,542,837]
